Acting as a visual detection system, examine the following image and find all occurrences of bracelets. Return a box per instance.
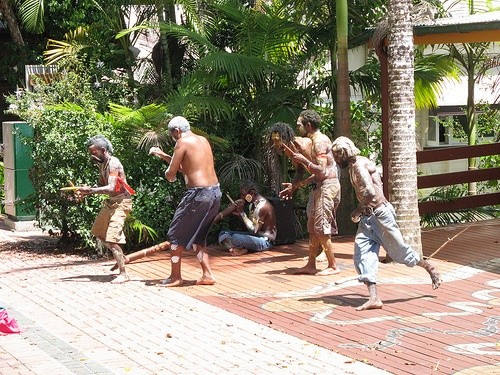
[294,182,297,190]
[307,161,313,171]
[300,181,303,188]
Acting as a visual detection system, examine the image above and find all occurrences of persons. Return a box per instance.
[76,135,133,285]
[270,122,340,263]
[147,116,222,288]
[218,179,277,258]
[330,136,444,311]
[293,110,341,277]
